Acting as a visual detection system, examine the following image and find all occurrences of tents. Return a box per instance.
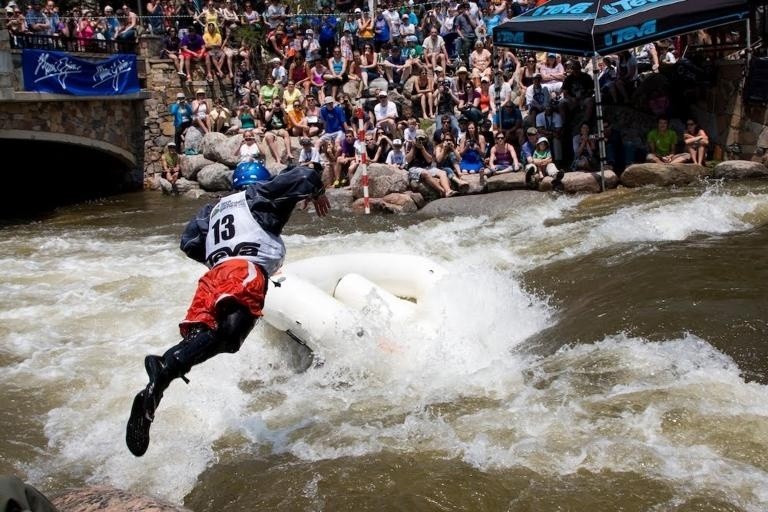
[488,0,768,191]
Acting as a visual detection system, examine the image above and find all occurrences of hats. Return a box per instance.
[272,8,556,146]
[196,88,206,94]
[176,92,185,99]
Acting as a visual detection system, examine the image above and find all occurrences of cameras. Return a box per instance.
[447,141,450,146]
[417,137,423,144]
[468,137,474,147]
[442,83,450,91]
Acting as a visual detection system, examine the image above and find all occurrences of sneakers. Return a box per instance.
[526,167,534,183]
[554,169,564,180]
[445,182,469,197]
[333,179,347,188]
[126,356,172,457]
[177,71,214,83]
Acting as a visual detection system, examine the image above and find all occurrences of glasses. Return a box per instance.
[687,123,693,125]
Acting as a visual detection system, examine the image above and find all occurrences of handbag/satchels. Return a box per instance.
[159,49,164,58]
[570,155,589,172]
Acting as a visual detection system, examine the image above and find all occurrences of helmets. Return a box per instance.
[232,161,272,191]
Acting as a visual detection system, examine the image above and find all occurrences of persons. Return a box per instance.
[126,161,330,456]
[1,1,145,58]
[141,1,743,197]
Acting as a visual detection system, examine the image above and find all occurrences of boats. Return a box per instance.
[260,252,446,364]
